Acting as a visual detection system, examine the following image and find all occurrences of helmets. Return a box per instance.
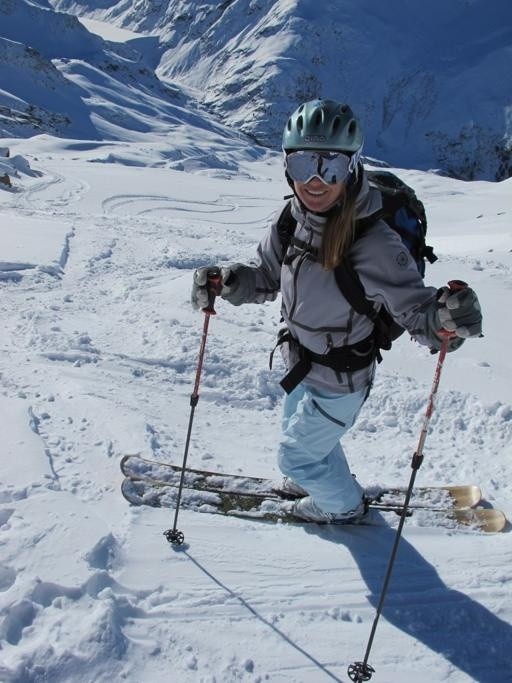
[280,97,363,152]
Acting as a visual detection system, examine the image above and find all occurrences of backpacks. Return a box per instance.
[274,162,438,353]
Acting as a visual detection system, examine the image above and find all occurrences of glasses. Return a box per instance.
[284,149,351,186]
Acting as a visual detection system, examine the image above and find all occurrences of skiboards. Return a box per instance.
[120,454,507,535]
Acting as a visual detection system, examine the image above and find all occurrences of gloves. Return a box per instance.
[190,264,231,313]
[427,278,485,340]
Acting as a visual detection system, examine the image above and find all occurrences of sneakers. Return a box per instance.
[296,495,373,525]
[272,472,306,497]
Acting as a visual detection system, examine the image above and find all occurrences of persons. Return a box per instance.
[190,97,484,522]
